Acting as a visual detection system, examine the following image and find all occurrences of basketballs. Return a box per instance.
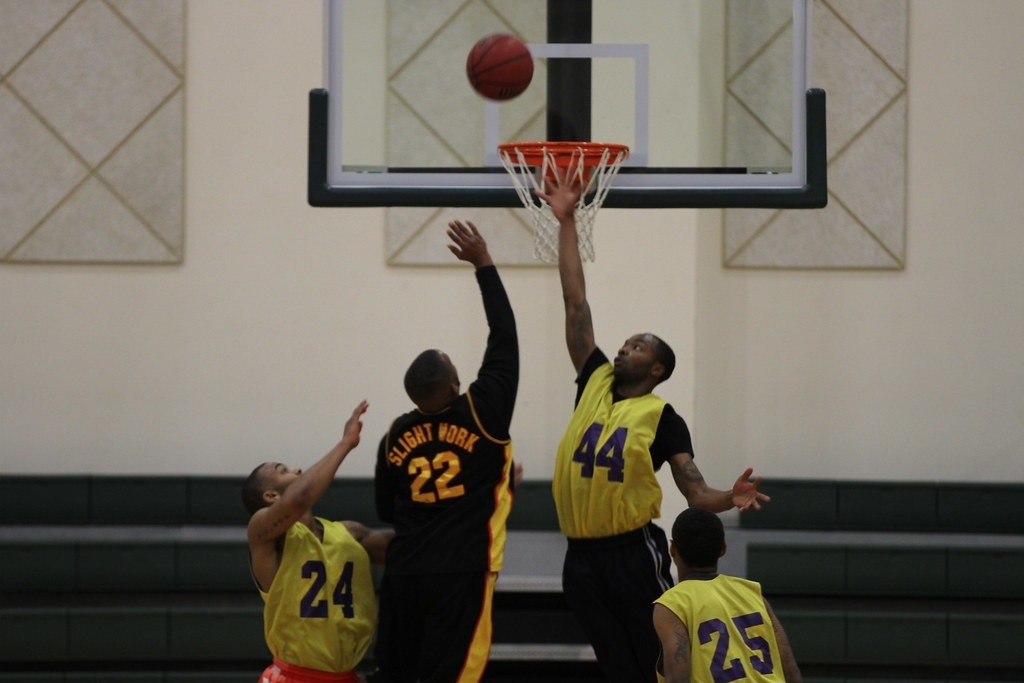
[466,31,535,101]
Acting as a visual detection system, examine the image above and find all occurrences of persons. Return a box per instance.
[532,165,772,683]
[245,399,524,683]
[652,507,802,683]
[372,221,524,682]
